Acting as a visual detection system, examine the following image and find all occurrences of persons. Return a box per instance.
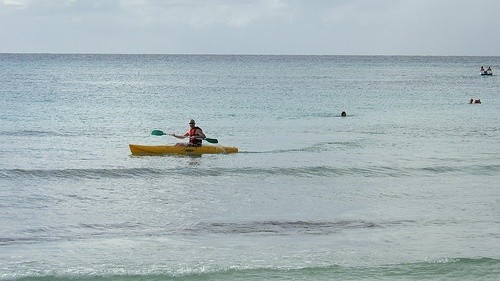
[481,66,491,70]
[174,118,206,146]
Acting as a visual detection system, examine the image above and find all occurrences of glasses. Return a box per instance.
[189,123,195,124]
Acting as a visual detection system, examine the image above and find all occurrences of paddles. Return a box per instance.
[151,130,218,143]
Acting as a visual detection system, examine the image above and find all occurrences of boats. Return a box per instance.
[128,144,239,158]
[480,70,493,76]
